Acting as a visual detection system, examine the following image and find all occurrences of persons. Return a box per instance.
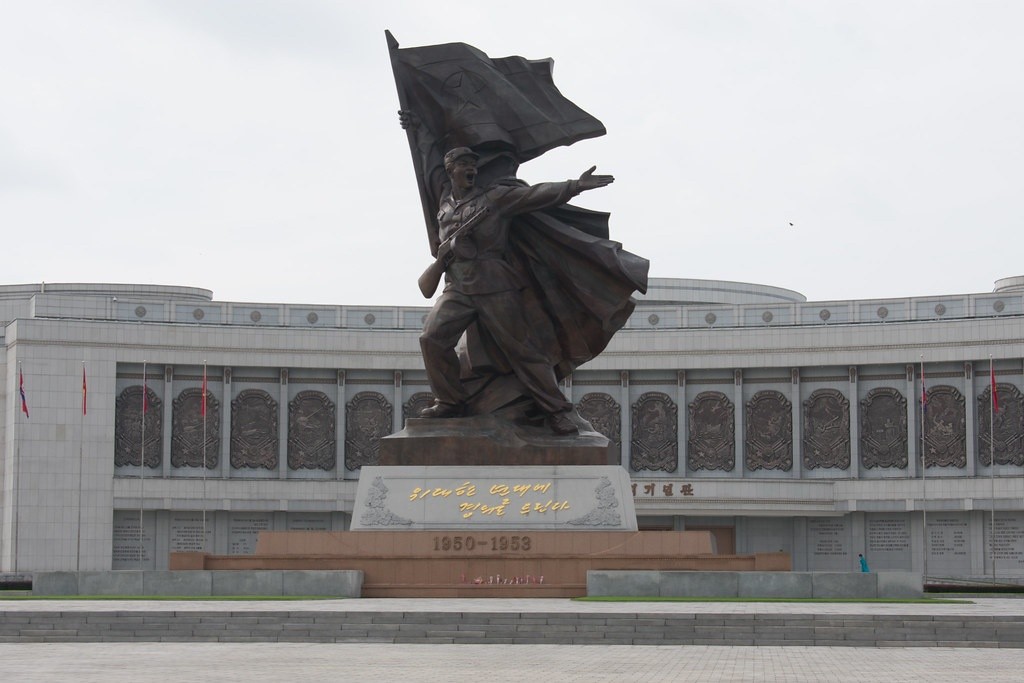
[993,405,1006,428]
[418,146,615,435]
[816,423,828,436]
[926,412,953,436]
[591,416,609,436]
[361,415,380,441]
[648,424,659,435]
[648,402,665,418]
[766,416,782,435]
[859,554,870,572]
[296,410,309,434]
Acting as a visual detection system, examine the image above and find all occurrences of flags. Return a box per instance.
[19,361,29,419]
[201,360,206,416]
[143,360,147,415]
[920,354,928,416]
[990,354,999,413]
[393,43,607,163]
[82,361,87,415]
